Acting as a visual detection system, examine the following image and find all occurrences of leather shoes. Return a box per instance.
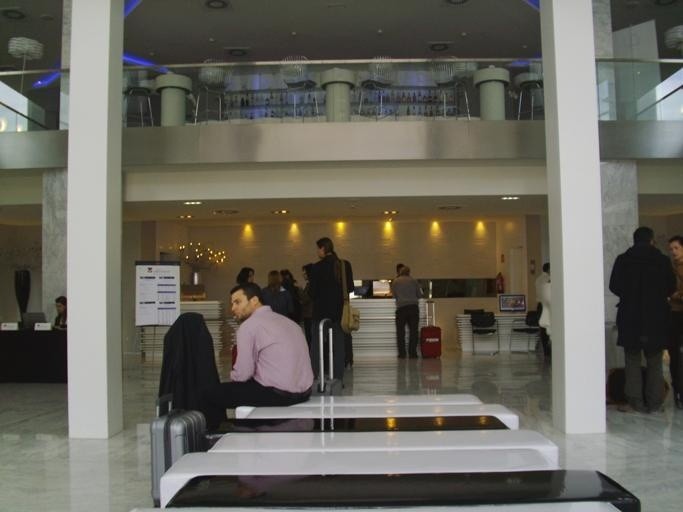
[617,401,666,414]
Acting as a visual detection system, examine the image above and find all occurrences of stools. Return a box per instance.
[515,78,544,121]
[354,78,391,118]
[192,81,228,124]
[283,78,320,122]
[131,87,155,125]
[434,79,473,122]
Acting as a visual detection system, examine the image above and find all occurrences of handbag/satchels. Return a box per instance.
[340,304,360,335]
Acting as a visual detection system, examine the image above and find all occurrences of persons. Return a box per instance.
[52,296,66,330]
[609,226,683,415]
[204,282,314,433]
[535,263,549,356]
[236,237,354,388]
[392,263,421,359]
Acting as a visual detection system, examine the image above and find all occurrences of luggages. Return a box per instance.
[420,300,442,359]
[311,317,343,397]
[150,394,208,508]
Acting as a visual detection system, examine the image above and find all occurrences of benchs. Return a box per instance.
[129,393,641,512]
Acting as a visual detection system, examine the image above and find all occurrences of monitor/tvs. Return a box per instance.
[500,295,526,311]
[349,279,362,299]
[22,312,46,329]
[373,280,392,296]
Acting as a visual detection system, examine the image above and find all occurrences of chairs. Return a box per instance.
[508,311,539,353]
[472,313,501,355]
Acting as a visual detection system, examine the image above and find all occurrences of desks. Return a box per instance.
[320,68,358,124]
[473,68,511,121]
[156,74,192,124]
